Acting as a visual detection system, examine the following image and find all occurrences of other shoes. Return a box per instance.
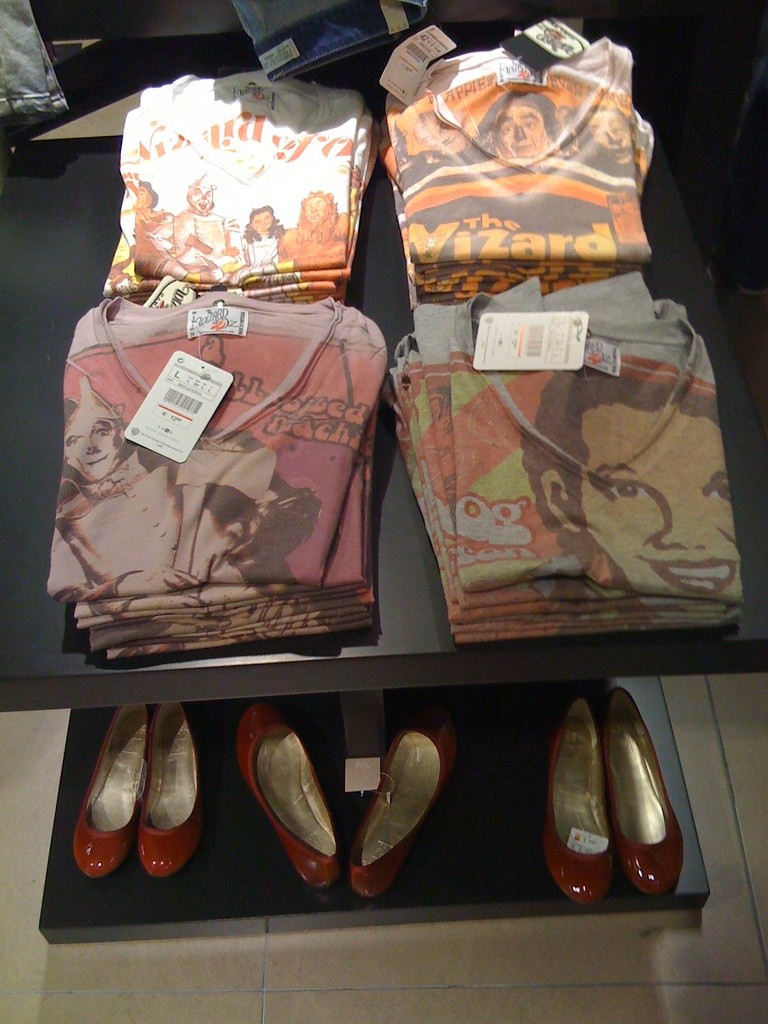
[350,705,457,897]
[138,702,203,878]
[712,242,768,296]
[74,705,148,878]
[237,701,341,890]
[602,687,683,896]
[542,697,614,904]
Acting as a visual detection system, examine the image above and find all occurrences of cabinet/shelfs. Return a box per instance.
[1,116,768,946]
